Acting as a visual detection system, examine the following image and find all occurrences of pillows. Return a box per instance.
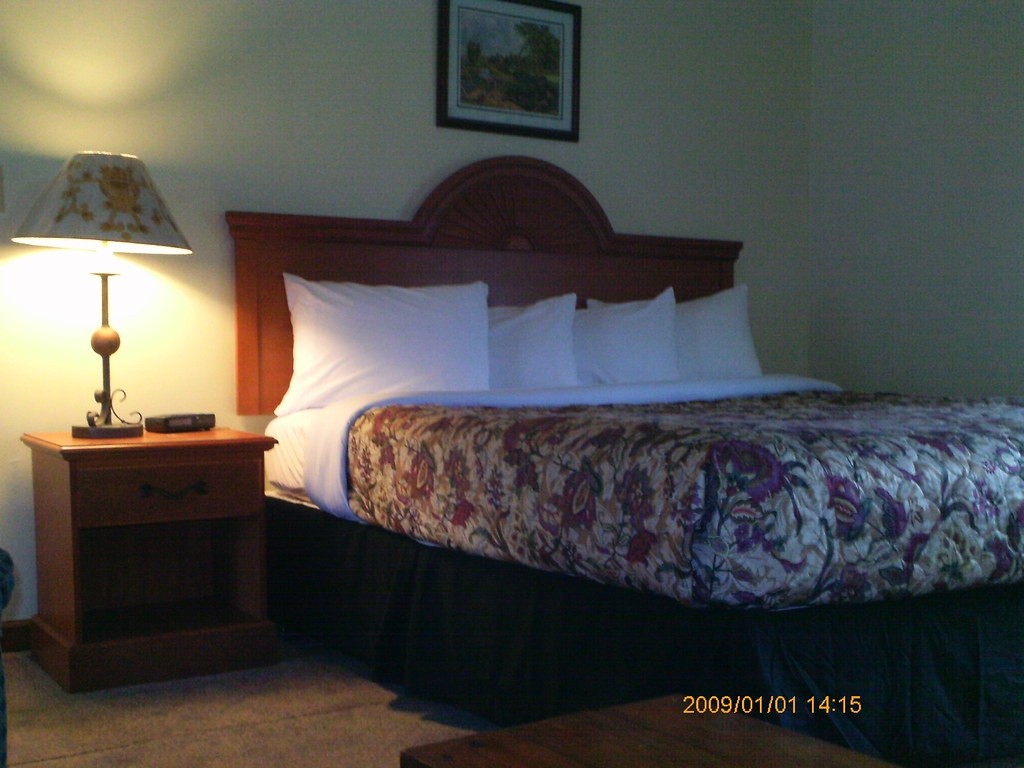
[273,272,488,417]
[586,279,764,380]
[575,286,683,387]
[488,292,579,391]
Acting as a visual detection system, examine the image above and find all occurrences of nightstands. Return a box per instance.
[20,424,280,695]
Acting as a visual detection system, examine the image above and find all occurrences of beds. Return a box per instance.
[227,154,1024,767]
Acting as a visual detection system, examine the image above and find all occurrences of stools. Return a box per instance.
[402,693,895,768]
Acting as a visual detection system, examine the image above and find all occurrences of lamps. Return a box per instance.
[9,153,193,440]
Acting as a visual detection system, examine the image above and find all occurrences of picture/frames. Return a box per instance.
[436,0,583,142]
